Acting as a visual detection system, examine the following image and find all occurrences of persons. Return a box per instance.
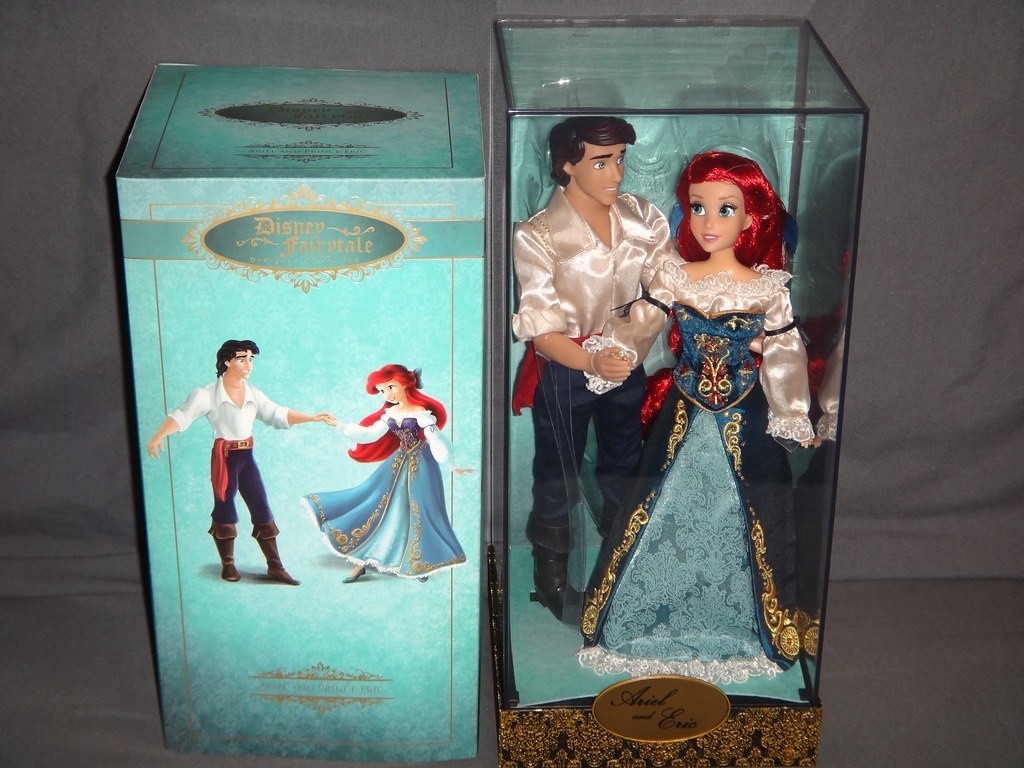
[578,144,817,685]
[510,115,764,631]
